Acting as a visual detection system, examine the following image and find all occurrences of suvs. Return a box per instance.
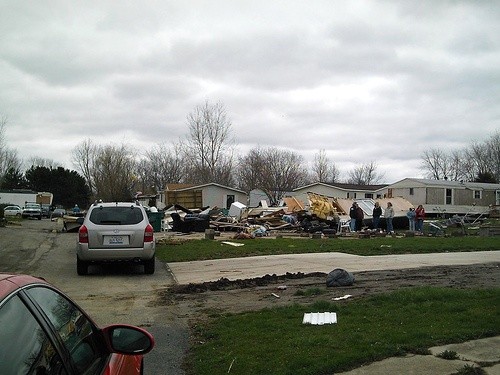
[77,199,155,274]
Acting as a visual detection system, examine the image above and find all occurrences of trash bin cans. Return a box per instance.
[145,210,164,233]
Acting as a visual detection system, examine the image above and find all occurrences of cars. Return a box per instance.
[0,272,154,375]
[4,202,67,221]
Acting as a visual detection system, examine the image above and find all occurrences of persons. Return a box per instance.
[384,202,395,234]
[372,202,382,230]
[349,202,364,233]
[74,204,80,212]
[416,205,425,230]
[407,208,416,231]
